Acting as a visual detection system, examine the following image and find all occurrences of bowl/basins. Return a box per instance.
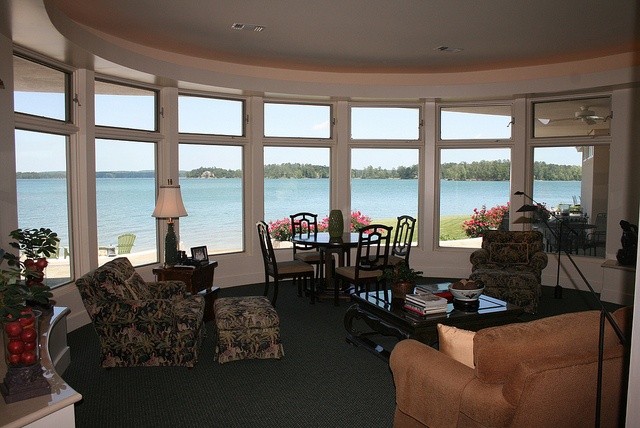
[448,283,485,302]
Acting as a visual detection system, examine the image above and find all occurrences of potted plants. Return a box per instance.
[2,251,44,329]
[383,259,425,300]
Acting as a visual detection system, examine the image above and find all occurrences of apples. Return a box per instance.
[24,259,33,266]
[29,264,39,273]
[33,282,43,288]
[19,311,35,327]
[20,351,37,365]
[23,341,37,351]
[35,263,42,270]
[8,354,20,364]
[37,258,47,267]
[34,277,43,282]
[26,278,35,287]
[7,341,24,354]
[22,328,36,343]
[4,322,21,336]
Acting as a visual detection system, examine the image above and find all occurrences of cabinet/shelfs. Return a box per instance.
[601,259,635,308]
[152,257,218,296]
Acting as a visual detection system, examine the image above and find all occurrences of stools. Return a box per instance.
[217,296,281,360]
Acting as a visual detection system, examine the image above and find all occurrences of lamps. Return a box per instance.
[150,185,188,264]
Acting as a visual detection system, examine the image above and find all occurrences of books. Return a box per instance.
[404,292,448,320]
[414,284,456,303]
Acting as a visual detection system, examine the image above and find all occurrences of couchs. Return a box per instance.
[472,231,548,314]
[75,257,205,369]
[388,307,639,428]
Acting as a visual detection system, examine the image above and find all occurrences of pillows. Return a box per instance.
[126,271,148,301]
[489,241,528,264]
[435,321,474,369]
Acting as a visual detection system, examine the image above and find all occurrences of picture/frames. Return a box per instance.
[190,246,208,260]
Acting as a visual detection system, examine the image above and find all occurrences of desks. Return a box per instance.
[1,306,85,428]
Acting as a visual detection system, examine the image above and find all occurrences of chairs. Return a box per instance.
[290,213,334,281]
[117,233,136,256]
[335,224,393,306]
[587,212,611,240]
[366,216,417,267]
[256,220,316,305]
[556,219,576,254]
[44,237,61,259]
